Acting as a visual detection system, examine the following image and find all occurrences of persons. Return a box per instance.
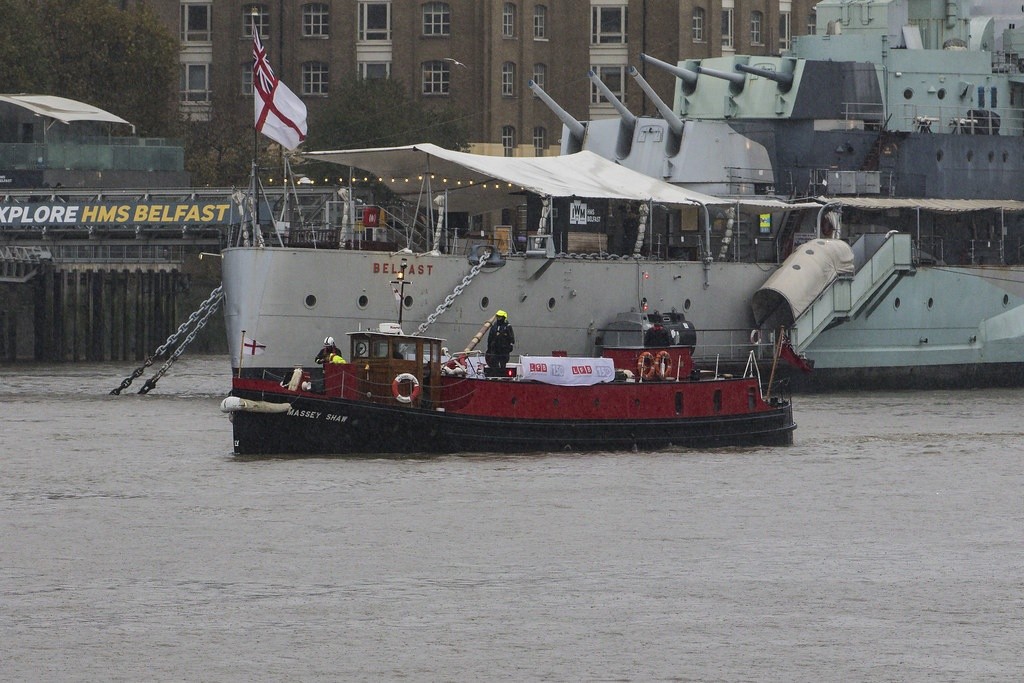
[392,344,404,359]
[314,337,341,370]
[487,309,516,376]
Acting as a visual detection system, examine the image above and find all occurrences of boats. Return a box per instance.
[220,256,797,455]
[781,339,815,373]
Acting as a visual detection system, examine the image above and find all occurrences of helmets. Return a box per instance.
[323,336,335,347]
[495,309,508,320]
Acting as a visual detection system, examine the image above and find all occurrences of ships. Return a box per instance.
[199,0,1024,389]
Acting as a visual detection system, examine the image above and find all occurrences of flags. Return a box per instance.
[779,334,813,375]
[243,337,266,356]
[252,25,307,151]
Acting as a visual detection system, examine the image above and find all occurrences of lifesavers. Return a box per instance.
[391,372,420,404]
[637,350,673,382]
